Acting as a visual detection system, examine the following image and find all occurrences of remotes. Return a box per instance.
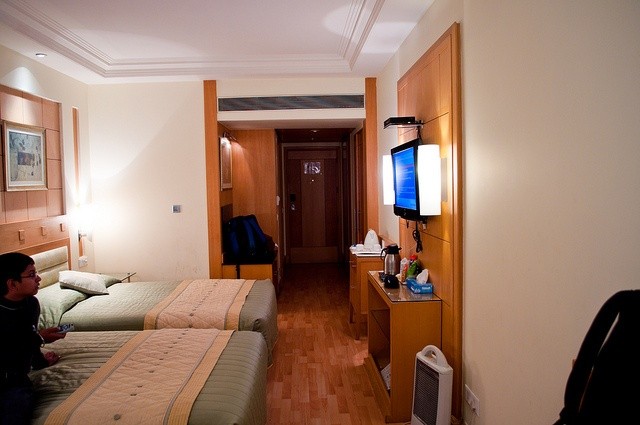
[56,323,75,333]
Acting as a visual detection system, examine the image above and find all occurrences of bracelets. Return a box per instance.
[36,331,45,347]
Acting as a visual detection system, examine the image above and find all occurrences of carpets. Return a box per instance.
[327,263,350,283]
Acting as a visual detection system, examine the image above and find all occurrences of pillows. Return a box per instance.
[58,270,109,296]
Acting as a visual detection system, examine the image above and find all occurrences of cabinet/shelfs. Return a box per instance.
[362,270,442,424]
[348,245,383,340]
[221,249,279,302]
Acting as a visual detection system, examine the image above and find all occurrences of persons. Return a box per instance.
[0,251,65,424]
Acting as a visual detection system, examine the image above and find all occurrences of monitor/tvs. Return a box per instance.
[390,138,424,220]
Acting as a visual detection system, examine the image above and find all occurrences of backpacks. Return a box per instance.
[554,289,636,424]
[223,214,275,278]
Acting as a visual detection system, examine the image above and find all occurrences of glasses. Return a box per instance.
[12,270,37,279]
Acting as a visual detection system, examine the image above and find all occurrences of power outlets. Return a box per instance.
[171,203,182,213]
[464,387,481,416]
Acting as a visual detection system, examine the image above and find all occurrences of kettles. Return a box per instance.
[380,242,402,277]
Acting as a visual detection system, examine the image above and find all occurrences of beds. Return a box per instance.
[27,329,268,425]
[0,239,279,370]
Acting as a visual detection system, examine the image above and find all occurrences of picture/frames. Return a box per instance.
[1,119,49,192]
[219,136,233,192]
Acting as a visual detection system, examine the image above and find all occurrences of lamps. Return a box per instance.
[417,144,443,216]
